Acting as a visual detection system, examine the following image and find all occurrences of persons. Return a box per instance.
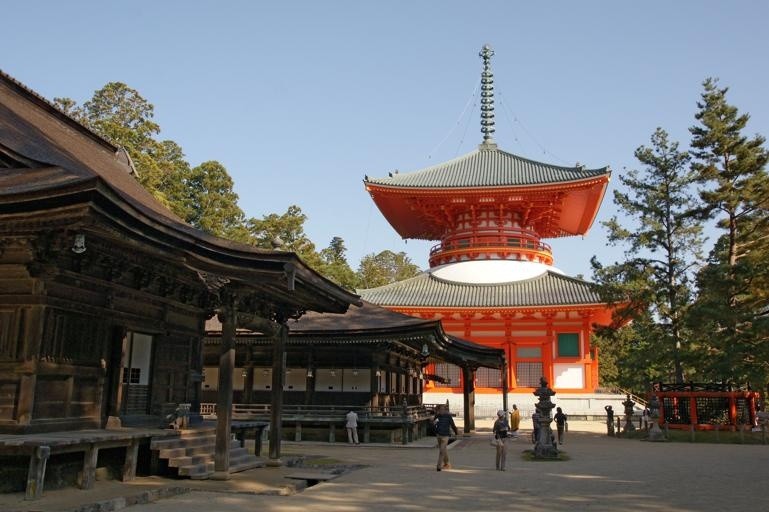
[532,408,542,444]
[344,407,361,445]
[492,410,512,470]
[508,405,520,442]
[554,407,567,446]
[430,404,461,472]
[604,405,615,436]
[642,404,650,431]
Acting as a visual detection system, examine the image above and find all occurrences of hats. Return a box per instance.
[496,410,506,418]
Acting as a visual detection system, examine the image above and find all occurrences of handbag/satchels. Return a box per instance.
[564,422,567,432]
[490,435,503,447]
